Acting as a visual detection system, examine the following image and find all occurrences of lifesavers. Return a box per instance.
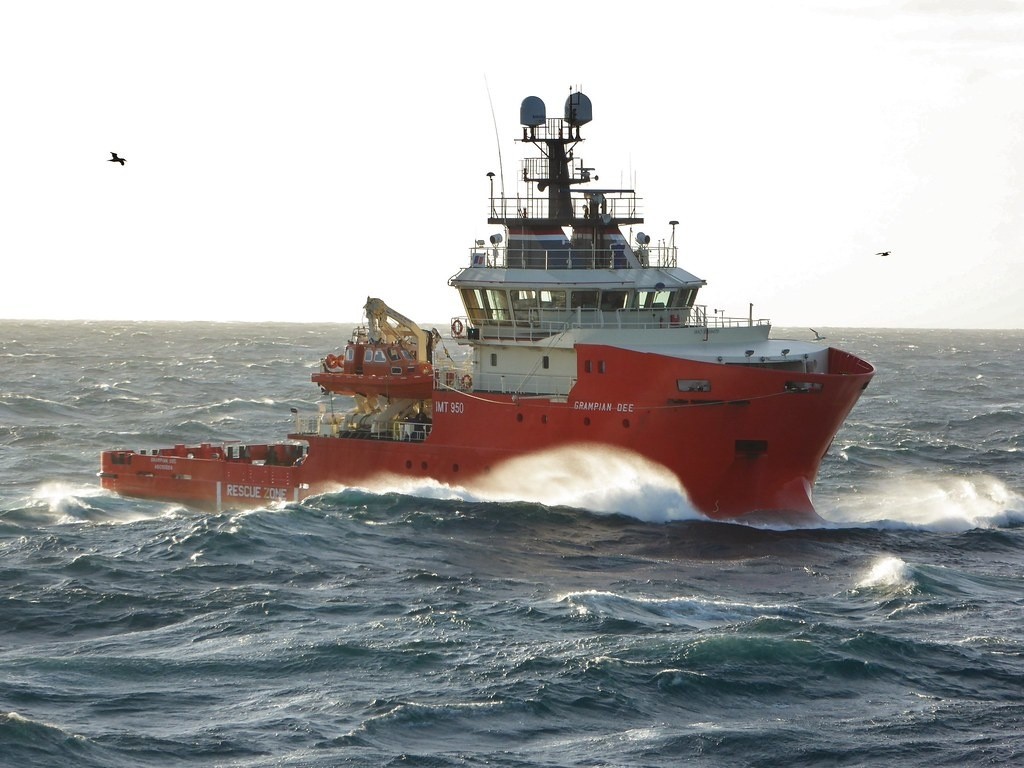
[451,320,463,335]
[336,354,345,368]
[326,353,338,368]
[461,373,473,390]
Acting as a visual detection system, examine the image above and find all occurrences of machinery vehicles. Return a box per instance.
[329,297,454,442]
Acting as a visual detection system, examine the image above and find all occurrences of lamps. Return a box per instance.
[781,348,789,359]
[486,171,496,180]
[490,234,503,247]
[745,349,755,357]
[635,232,651,248]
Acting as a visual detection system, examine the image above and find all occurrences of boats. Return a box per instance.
[308,325,472,399]
[96,68,876,517]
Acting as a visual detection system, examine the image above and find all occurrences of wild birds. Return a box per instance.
[875,251,891,256]
[107,152,127,166]
